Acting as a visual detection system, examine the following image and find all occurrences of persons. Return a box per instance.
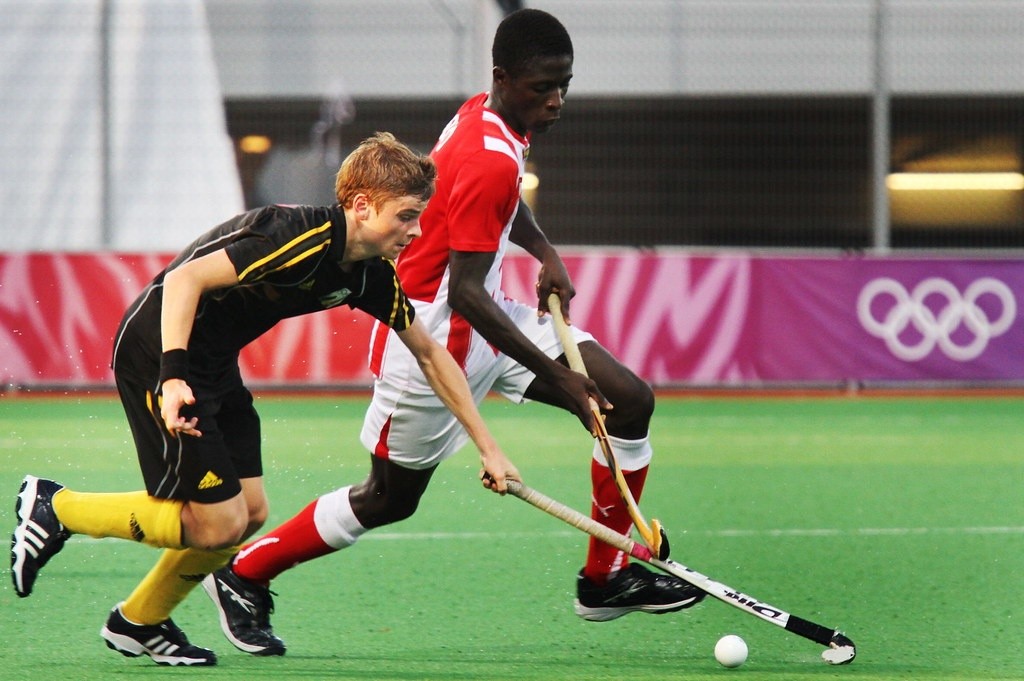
[193,7,708,654]
[10,128,523,673]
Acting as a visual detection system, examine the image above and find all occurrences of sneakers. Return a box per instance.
[98,602,217,665]
[9,476,70,597]
[200,553,286,655]
[574,564,704,622]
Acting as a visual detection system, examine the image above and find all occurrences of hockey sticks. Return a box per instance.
[547,293,671,563]
[479,466,858,665]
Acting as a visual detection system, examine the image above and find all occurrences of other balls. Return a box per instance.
[714,635,748,668]
